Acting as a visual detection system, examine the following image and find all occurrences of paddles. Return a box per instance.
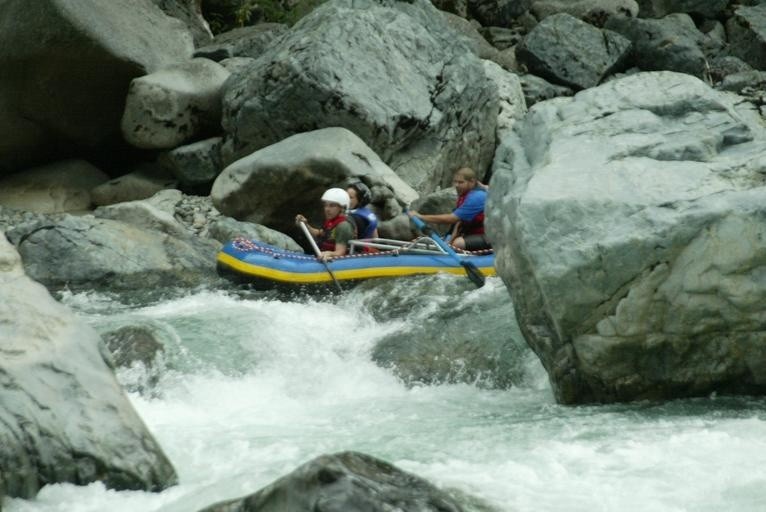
[406,208,484,288]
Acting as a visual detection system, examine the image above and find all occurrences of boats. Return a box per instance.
[213,235,497,296]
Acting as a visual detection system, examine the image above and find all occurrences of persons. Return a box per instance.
[341,182,382,256]
[295,188,353,260]
[408,166,492,253]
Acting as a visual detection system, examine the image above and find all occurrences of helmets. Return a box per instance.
[321,188,350,210]
[347,182,372,206]
